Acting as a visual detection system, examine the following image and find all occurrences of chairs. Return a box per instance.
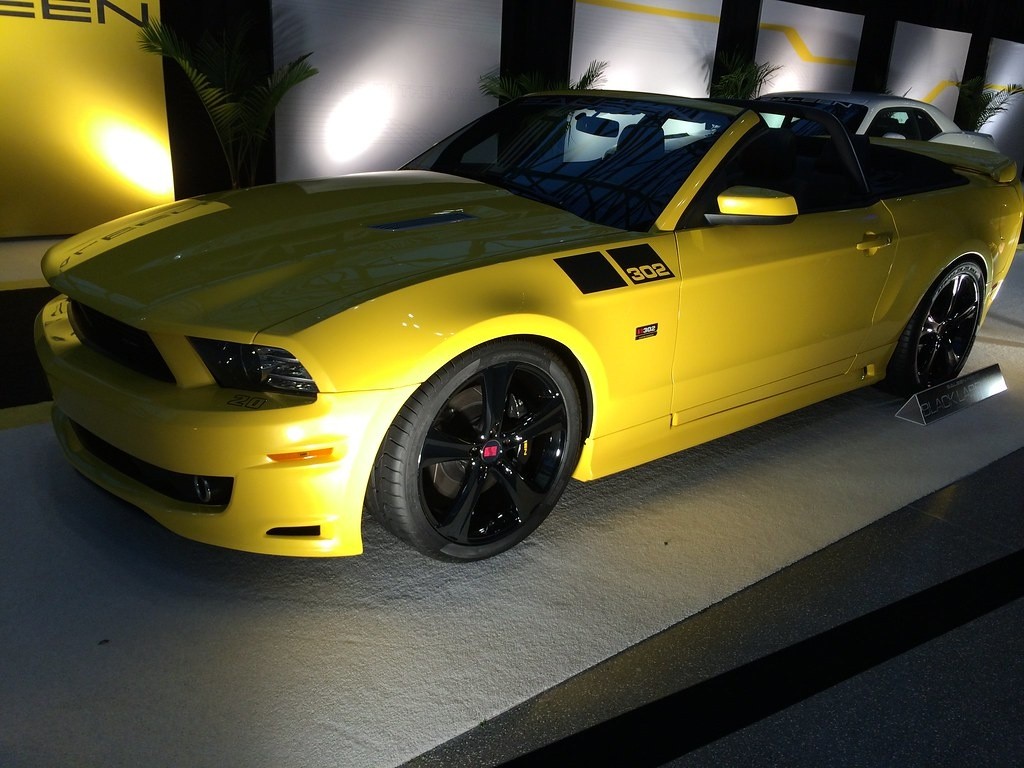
[582,127,809,231]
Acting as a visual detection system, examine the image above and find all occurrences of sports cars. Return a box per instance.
[603,88,1002,156]
[36,89,1024,565]
